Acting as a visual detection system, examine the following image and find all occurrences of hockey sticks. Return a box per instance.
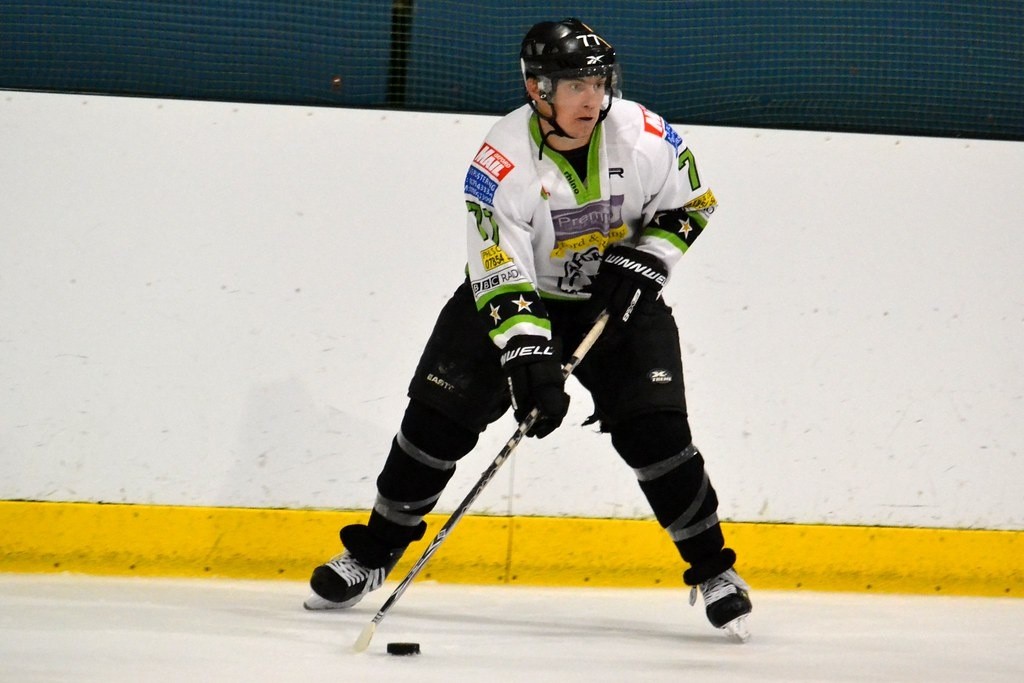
[354,307,609,651]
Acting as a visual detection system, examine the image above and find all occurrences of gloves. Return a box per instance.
[578,243,669,353]
[499,334,570,439]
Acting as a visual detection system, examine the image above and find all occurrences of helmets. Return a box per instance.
[519,19,617,96]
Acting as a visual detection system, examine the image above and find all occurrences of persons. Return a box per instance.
[303,16,753,645]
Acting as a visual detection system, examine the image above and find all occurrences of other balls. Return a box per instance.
[387,642,419,655]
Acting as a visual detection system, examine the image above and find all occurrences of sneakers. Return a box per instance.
[687,566,753,641]
[303,524,410,611]
